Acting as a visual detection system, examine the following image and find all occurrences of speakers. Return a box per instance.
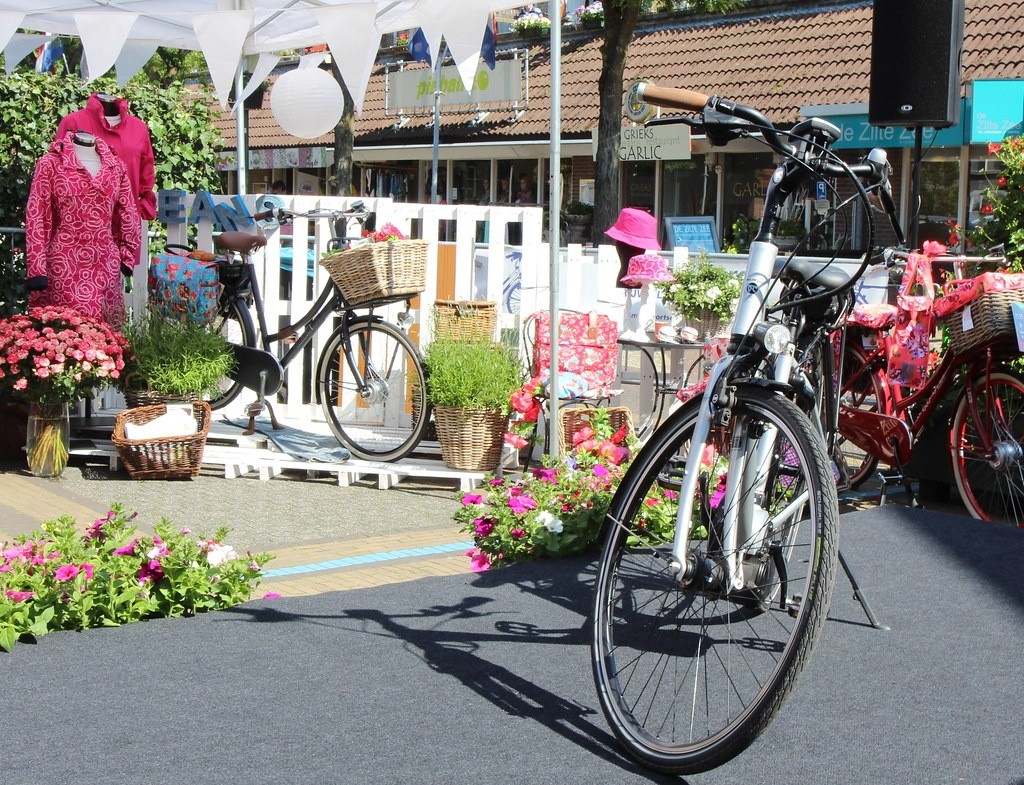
[869,0,966,132]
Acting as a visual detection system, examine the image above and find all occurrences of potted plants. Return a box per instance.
[228,71,274,110]
[776,218,807,245]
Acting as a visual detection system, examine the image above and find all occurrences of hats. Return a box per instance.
[603,208,663,251]
[620,254,677,287]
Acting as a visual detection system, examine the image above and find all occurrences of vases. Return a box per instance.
[582,16,604,30]
[908,406,1024,497]
[27,401,71,478]
[517,27,549,38]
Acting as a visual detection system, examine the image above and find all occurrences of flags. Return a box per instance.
[29,28,72,78]
[408,26,431,64]
[479,13,498,71]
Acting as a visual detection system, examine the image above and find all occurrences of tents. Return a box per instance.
[0,0,564,468]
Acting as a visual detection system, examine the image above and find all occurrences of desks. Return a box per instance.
[616,339,705,457]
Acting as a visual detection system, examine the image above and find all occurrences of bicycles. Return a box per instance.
[589,80,1023,776]
[143,201,433,462]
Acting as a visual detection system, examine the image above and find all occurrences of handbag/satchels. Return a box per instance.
[530,308,618,397]
[885,251,932,390]
[147,244,253,326]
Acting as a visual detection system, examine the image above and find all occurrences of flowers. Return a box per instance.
[508,12,551,34]
[454,406,795,574]
[319,221,407,257]
[506,375,547,447]
[572,0,605,22]
[910,131,1024,430]
[396,32,410,46]
[654,247,746,323]
[0,305,136,475]
[0,502,282,653]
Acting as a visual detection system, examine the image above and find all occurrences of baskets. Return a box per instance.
[558,406,637,466]
[318,236,428,306]
[433,297,499,342]
[111,402,211,480]
[934,273,1024,358]
[685,307,733,339]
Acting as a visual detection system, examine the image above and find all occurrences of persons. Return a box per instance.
[516,2,545,27]
[475,178,532,241]
[28,132,141,425]
[425,194,449,240]
[265,181,285,195]
[51,92,158,242]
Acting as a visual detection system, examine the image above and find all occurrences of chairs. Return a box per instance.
[524,308,624,472]
